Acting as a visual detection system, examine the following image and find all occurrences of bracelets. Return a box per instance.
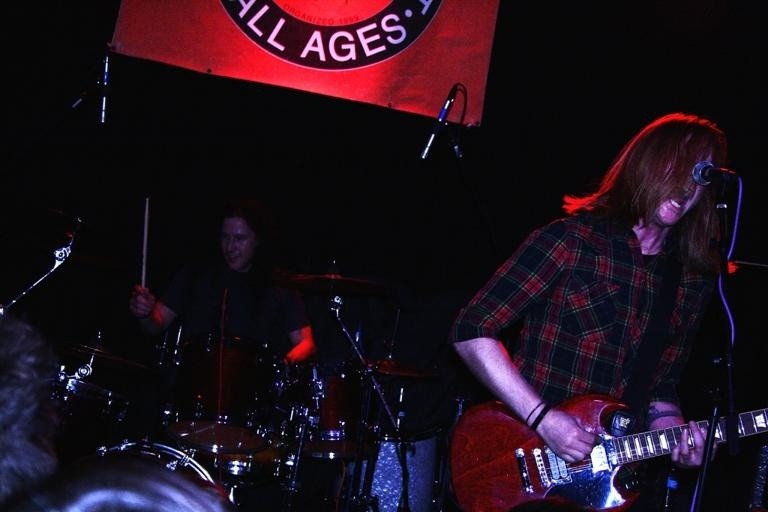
[525,397,552,431]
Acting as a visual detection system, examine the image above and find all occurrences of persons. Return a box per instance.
[128,195,317,366]
[445,111,729,512]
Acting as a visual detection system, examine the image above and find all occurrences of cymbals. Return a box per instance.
[342,361,436,376]
[63,344,143,367]
[176,420,272,455]
[288,273,384,284]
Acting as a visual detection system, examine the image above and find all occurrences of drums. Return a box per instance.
[343,424,441,511]
[60,442,230,512]
[277,363,368,462]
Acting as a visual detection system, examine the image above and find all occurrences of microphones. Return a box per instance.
[692,160,736,186]
[419,84,458,160]
[99,55,112,124]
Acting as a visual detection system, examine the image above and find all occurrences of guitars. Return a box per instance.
[452,393,768,512]
